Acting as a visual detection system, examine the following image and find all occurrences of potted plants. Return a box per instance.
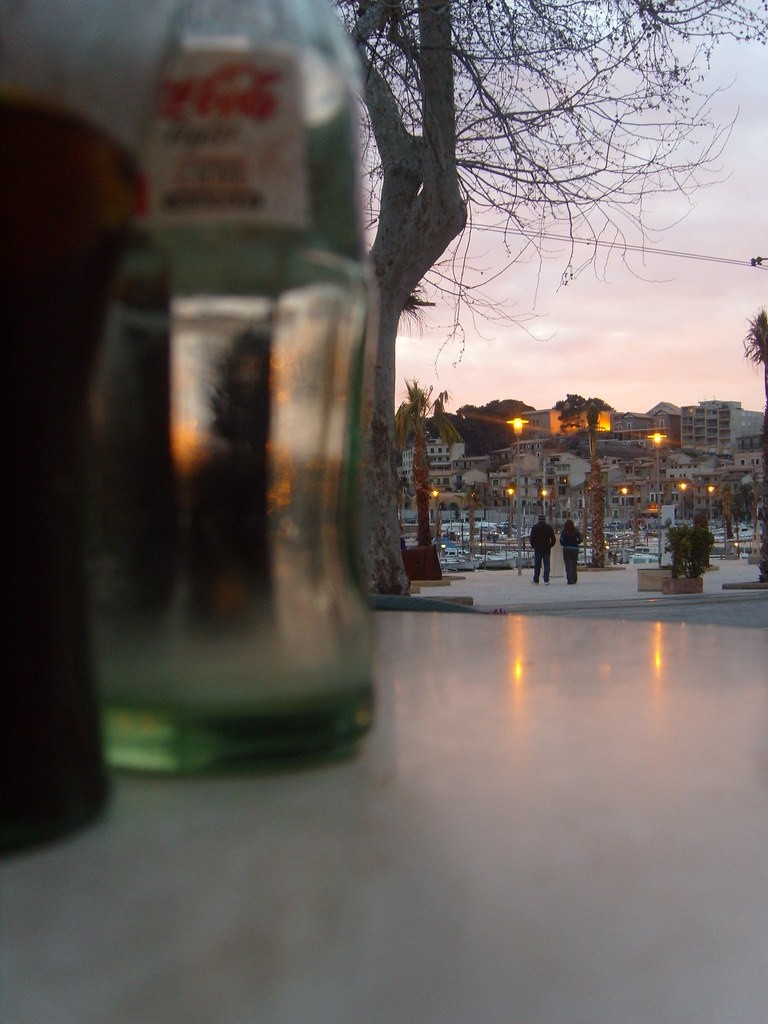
[662,522,716,595]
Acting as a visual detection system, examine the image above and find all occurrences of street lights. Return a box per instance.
[647,431,668,566]
[506,487,516,538]
[677,482,688,524]
[432,490,441,555]
[619,486,629,564]
[506,417,529,576]
[708,485,715,533]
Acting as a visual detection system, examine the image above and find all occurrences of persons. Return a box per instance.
[559,519,583,585]
[530,515,556,585]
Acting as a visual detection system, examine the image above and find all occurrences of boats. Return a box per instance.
[397,514,763,571]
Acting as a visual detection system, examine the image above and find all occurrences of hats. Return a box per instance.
[538,514,546,520]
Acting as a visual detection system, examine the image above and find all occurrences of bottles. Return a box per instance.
[101,0,375,773]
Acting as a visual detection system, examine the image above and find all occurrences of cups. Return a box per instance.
[0,0,174,862]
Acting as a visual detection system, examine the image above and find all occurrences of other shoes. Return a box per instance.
[544,580,551,585]
[532,577,539,585]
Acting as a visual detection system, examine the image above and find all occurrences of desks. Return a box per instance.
[1,587,768,1024]
